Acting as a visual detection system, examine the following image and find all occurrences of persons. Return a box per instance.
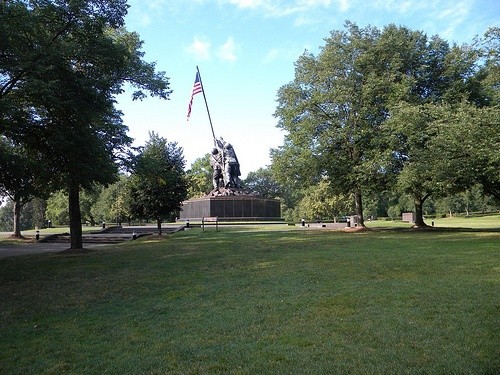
[216,137,242,186]
[210,148,225,190]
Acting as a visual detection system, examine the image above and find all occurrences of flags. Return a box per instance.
[186,71,203,121]
